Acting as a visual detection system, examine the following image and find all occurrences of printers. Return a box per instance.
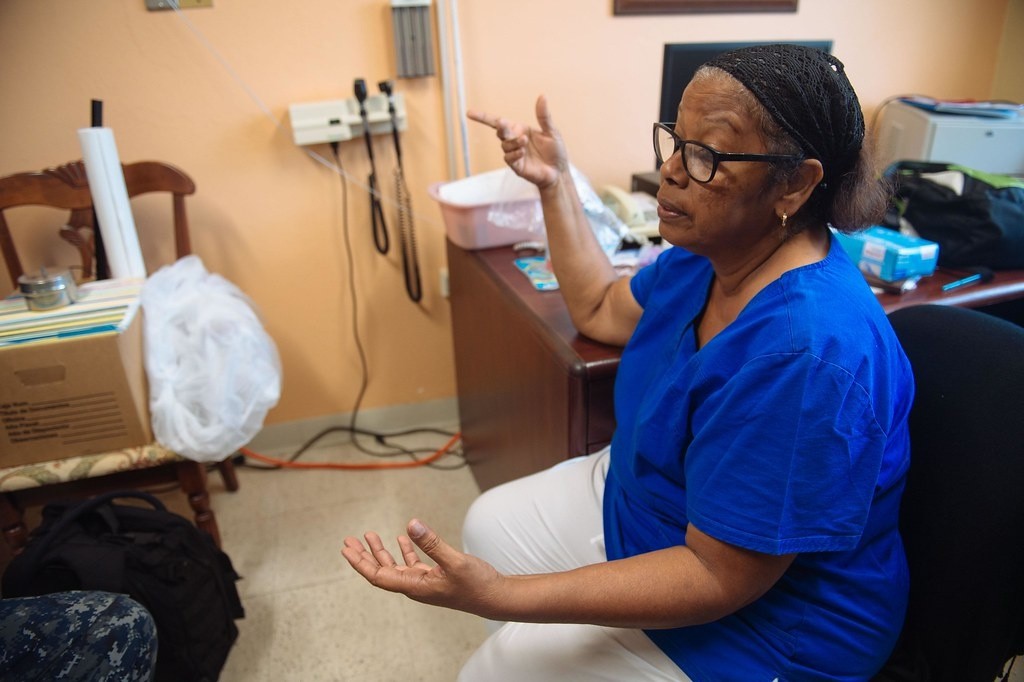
[873,98,1024,181]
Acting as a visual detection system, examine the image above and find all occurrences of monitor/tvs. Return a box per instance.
[655,39,832,170]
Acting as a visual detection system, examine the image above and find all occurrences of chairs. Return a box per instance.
[886,303,1024,682]
[0,158,241,551]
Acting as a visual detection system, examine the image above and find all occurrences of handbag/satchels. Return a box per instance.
[1,489,246,682]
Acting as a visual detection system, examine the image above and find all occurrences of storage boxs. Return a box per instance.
[0,278,152,468]
[425,169,544,247]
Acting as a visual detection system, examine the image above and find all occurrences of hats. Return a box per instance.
[706,44,867,182]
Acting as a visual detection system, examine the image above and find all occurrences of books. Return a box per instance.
[897,96,1024,119]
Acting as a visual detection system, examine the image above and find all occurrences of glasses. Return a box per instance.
[652,122,793,183]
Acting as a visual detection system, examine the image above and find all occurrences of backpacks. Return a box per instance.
[875,160,1024,270]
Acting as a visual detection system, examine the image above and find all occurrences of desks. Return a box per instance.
[445,244,1024,493]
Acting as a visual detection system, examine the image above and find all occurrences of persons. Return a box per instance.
[1,591,157,682]
[341,44,915,682]
